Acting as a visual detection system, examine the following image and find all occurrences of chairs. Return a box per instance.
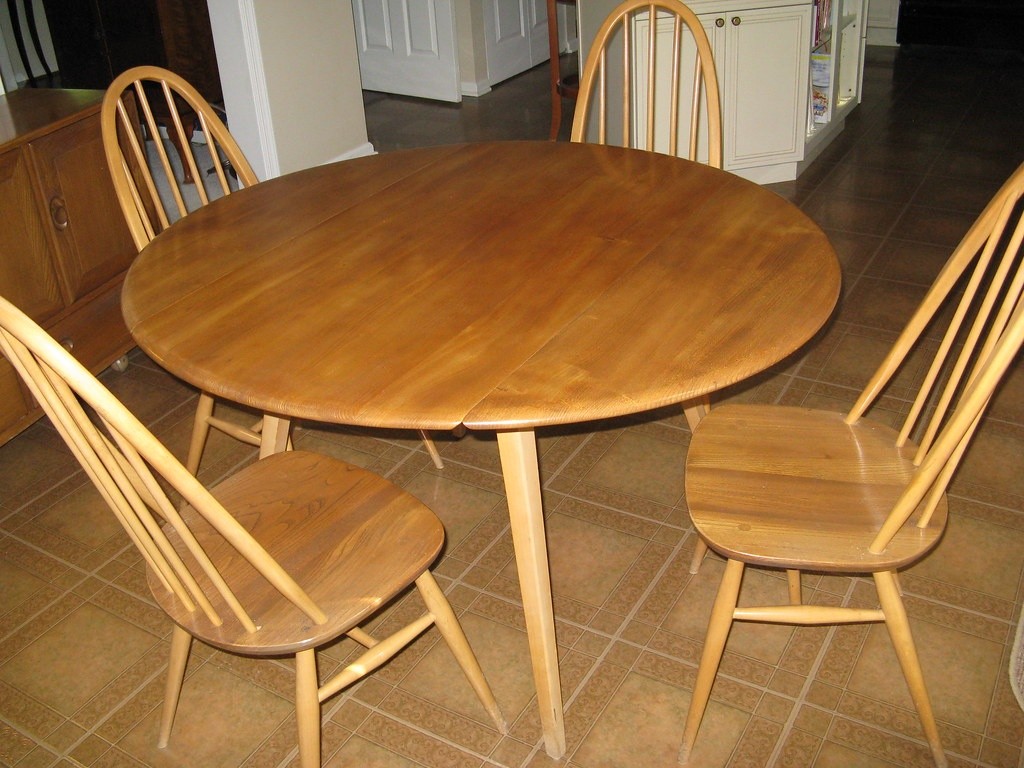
[671,153,1024,768]
[0,292,514,767]
[567,0,731,434]
[97,65,448,480]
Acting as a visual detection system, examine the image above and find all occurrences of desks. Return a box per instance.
[119,134,849,762]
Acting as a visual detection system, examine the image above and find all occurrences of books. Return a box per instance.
[805,0,834,137]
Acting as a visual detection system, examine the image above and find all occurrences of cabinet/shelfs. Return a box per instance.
[352,0,579,107]
[577,0,902,185]
[0,85,168,452]
